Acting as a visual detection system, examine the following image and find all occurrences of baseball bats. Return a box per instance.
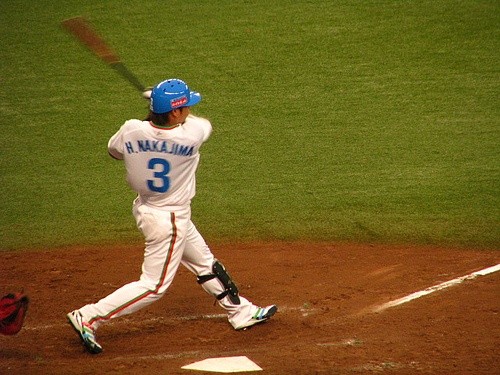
[62,14,151,103]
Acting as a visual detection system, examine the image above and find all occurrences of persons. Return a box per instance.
[66,79,278,354]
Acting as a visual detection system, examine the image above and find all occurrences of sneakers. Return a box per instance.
[235,305,277,330]
[67,310,102,353]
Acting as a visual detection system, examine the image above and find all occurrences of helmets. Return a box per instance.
[150,79,201,114]
